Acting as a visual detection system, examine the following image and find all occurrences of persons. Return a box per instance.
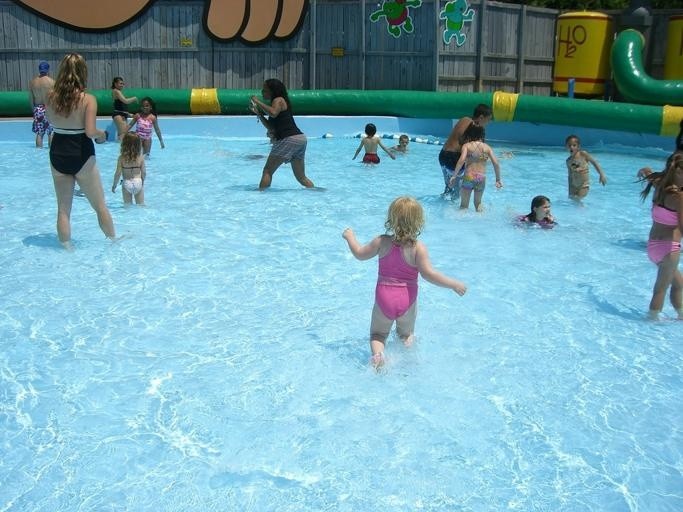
[45,50,116,247]
[28,62,58,147]
[564,134,609,200]
[635,153,683,321]
[522,195,554,223]
[117,97,166,159]
[111,131,148,206]
[448,120,504,212]
[109,75,138,138]
[248,79,314,191]
[342,195,468,367]
[351,121,396,164]
[390,135,411,153]
[438,103,493,202]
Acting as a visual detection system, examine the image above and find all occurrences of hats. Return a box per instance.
[39,63,49,73]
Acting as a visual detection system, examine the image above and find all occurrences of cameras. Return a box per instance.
[250,99,258,106]
